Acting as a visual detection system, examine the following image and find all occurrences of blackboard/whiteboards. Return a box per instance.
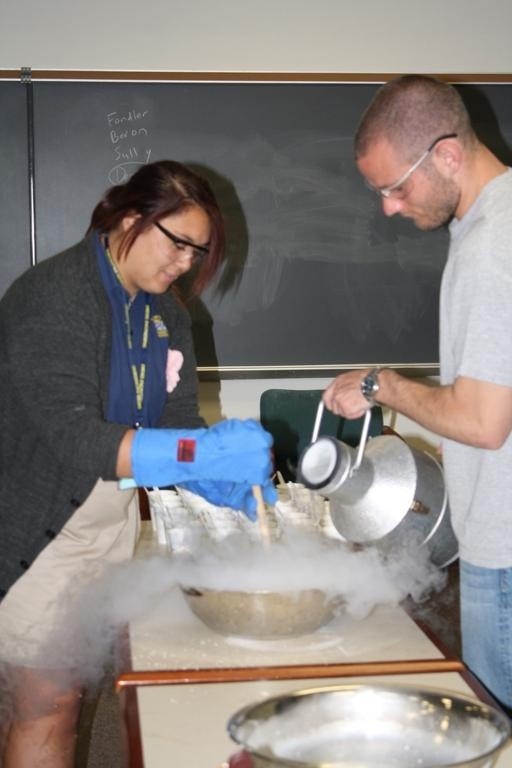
[0,70,512,381]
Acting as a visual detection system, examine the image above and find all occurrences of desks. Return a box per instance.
[101,479,512,768]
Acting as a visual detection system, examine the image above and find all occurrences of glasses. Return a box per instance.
[364,133,458,198]
[152,221,210,263]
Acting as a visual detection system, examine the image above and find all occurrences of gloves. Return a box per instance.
[131,417,275,487]
[176,477,277,523]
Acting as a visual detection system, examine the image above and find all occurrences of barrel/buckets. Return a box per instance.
[297,399,460,571]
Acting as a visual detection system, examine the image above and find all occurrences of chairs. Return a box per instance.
[260,389,386,488]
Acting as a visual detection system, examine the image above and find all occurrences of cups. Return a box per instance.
[145,480,353,553]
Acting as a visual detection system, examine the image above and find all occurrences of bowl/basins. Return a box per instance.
[177,577,345,643]
[227,681,511,767]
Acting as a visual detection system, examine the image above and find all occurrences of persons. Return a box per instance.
[320,76,512,720]
[1,157,280,767]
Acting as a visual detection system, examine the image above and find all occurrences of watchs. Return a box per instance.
[361,363,391,410]
